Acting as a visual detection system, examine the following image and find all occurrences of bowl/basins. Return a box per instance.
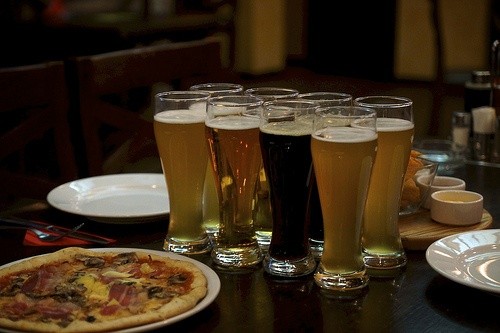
[416,175,466,209]
[430,189,483,226]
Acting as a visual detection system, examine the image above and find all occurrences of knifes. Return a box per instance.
[4,217,114,245]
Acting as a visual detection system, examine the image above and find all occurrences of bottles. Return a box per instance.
[451,112,470,154]
[464,71,490,114]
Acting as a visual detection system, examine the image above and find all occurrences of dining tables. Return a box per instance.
[0,129,500,333]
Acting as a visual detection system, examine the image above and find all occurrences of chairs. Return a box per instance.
[0,61,75,215]
[74,36,228,177]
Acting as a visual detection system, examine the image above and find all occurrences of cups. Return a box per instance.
[203,94,265,271]
[153,91,214,259]
[244,87,300,245]
[473,131,494,161]
[309,105,378,298]
[259,98,321,278]
[188,84,244,242]
[348,95,416,270]
[294,93,353,257]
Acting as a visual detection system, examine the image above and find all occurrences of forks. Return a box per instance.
[28,227,67,241]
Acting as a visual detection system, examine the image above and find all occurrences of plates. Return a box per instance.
[0,248,222,333]
[46,172,170,219]
[424,229,500,293]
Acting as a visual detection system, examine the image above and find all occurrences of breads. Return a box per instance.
[400,149,424,211]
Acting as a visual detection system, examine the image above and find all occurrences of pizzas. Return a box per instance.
[0,247,208,333]
